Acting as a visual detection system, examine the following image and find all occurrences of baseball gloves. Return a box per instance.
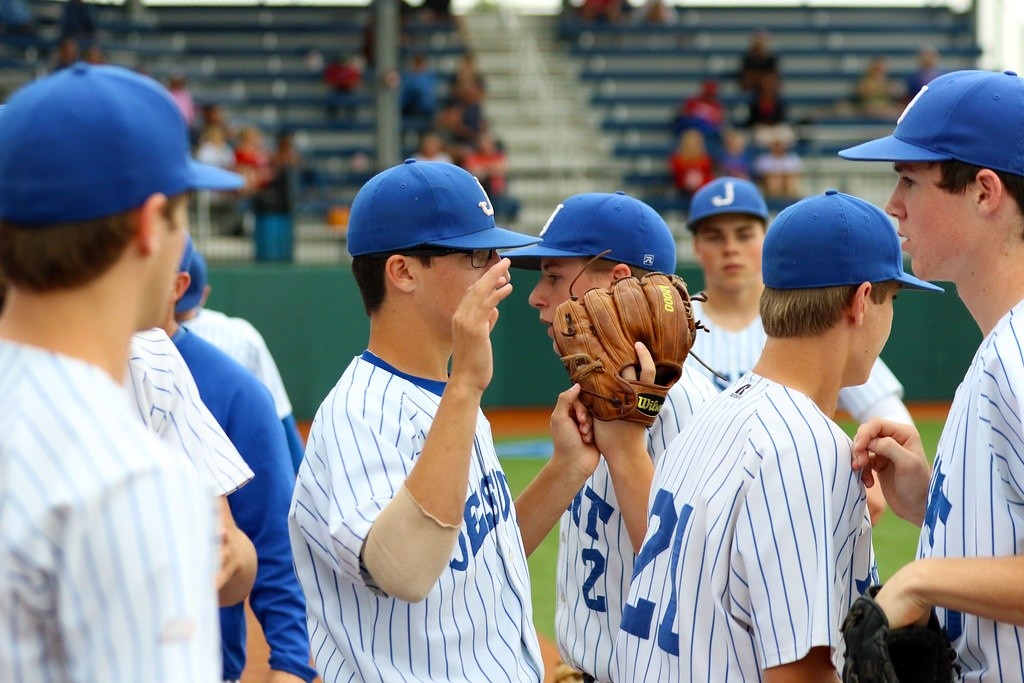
[839,584,958,683]
[550,247,712,430]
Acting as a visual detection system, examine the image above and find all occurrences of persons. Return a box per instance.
[840,69,1024,682]
[287,157,601,683]
[666,27,804,202]
[0,1,305,235]
[617,187,948,683]
[497,190,720,683]
[324,0,512,212]
[683,176,918,526]
[846,43,944,120]
[0,63,315,683]
[568,0,682,26]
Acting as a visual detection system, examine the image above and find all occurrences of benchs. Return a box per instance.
[0,0,986,265]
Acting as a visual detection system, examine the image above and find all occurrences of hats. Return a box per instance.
[176,239,207,313]
[497,189,678,275]
[1,63,243,226]
[837,69,1024,178]
[345,158,544,257]
[684,177,771,229]
[760,188,944,291]
